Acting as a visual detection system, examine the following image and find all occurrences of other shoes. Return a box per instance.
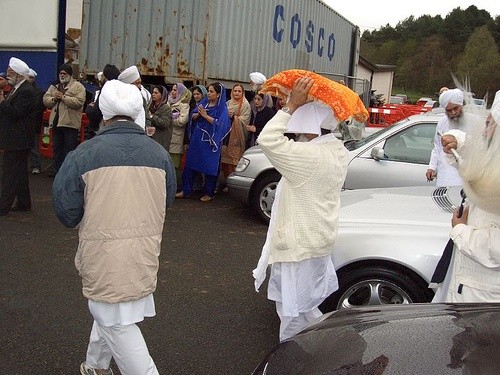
[176,191,184,198]
[200,195,215,202]
[214,188,221,193]
[31,168,40,174]
[10,201,31,211]
[79,360,114,375]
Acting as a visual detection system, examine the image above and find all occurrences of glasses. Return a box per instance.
[253,97,264,101]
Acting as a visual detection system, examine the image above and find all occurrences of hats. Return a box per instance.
[249,72,267,85]
[103,64,121,80]
[99,79,143,122]
[118,65,140,83]
[58,64,72,76]
[29,69,38,77]
[9,56,30,77]
[438,89,464,109]
[0,72,7,78]
[86,74,95,82]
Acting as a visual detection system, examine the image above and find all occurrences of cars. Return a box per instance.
[318,183,468,314]
[250,298,500,375]
[226,93,488,226]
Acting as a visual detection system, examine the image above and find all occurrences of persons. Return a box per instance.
[428,87,500,304]
[253,76,351,342]
[338,79,351,135]
[426,88,486,186]
[0,57,44,215]
[86,65,284,201]
[53,78,176,375]
[44,63,87,176]
